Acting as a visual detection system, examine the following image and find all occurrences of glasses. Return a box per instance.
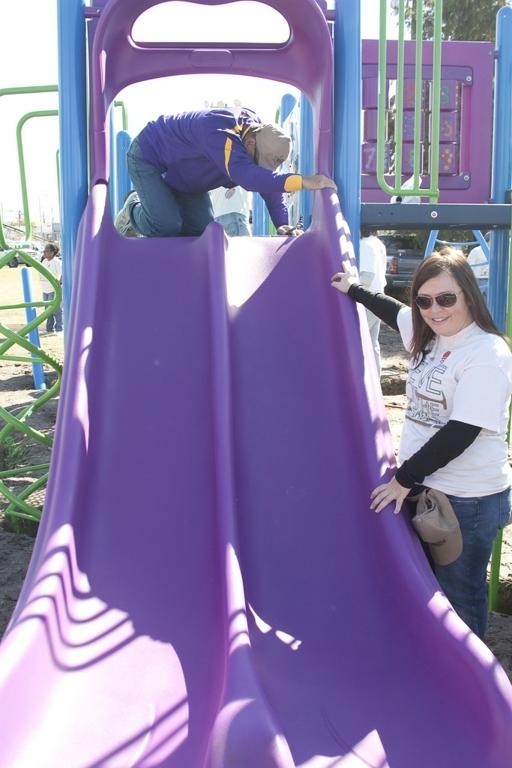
[414,287,465,310]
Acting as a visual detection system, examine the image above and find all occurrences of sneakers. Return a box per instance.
[113,188,142,237]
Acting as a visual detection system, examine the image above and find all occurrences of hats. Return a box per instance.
[251,122,292,172]
[403,486,465,567]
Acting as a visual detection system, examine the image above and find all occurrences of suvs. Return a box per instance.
[0,241,38,267]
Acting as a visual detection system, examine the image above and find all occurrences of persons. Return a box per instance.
[112,104,340,238]
[359,227,387,384]
[328,245,512,640]
[39,243,63,333]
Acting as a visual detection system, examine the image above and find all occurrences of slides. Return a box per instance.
[1,185,512,761]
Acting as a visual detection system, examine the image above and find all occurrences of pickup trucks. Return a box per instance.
[378,234,425,291]
